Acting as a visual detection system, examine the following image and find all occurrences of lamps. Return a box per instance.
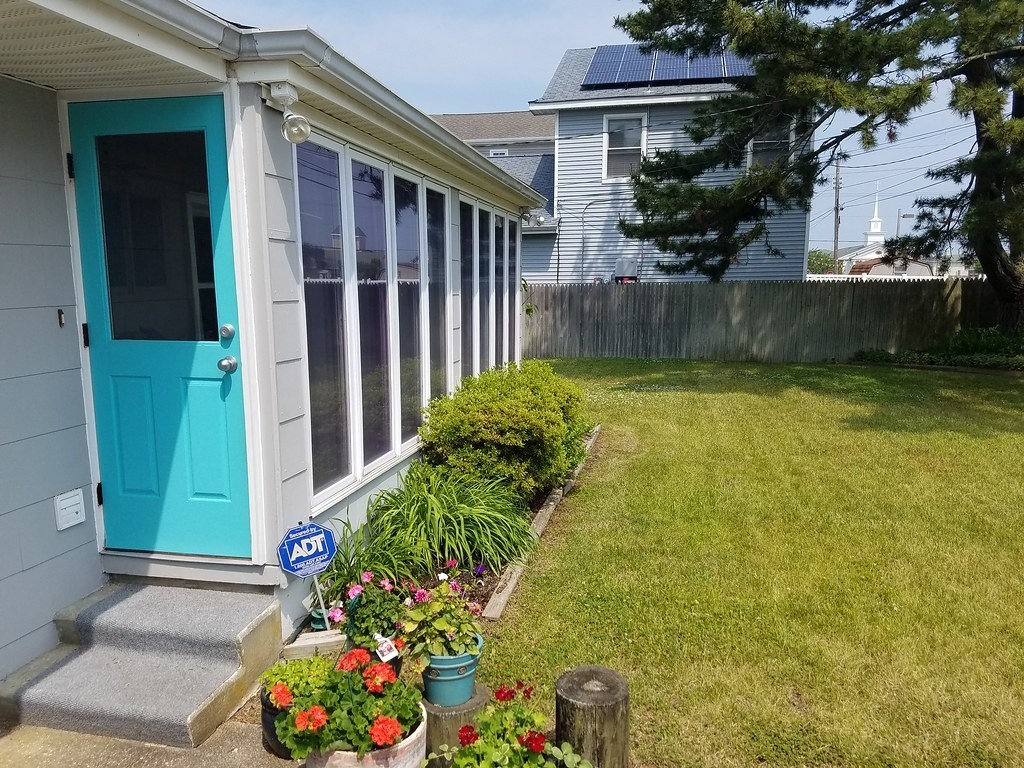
[280,103,311,145]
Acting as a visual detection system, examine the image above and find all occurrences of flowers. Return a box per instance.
[395,559,487,657]
[331,572,404,651]
[273,649,422,763]
[420,678,596,768]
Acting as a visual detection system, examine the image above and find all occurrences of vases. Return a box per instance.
[421,633,484,709]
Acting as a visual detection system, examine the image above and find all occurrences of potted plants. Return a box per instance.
[259,653,334,760]
